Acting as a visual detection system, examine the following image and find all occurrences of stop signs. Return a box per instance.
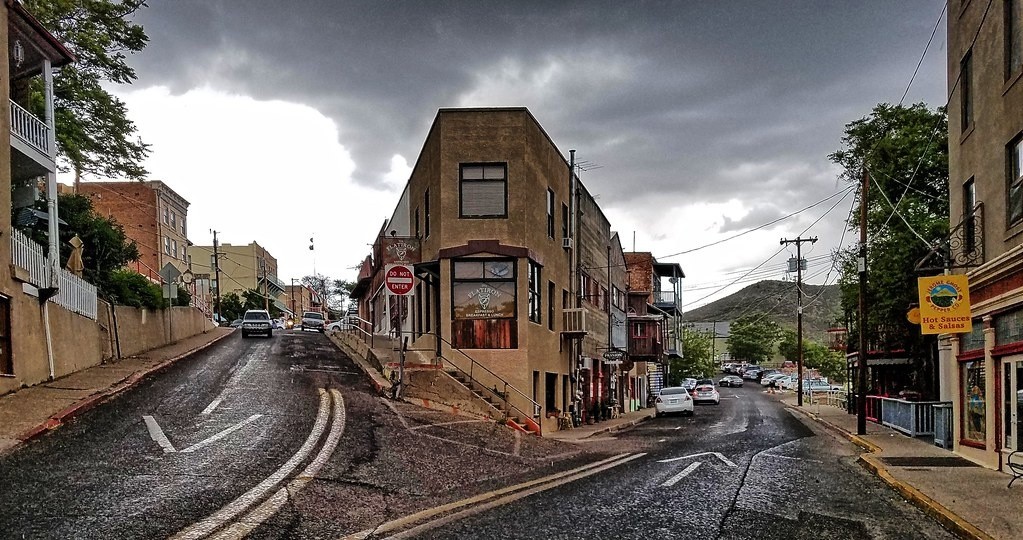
[386,266,413,294]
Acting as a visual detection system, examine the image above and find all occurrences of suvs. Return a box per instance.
[241,308,273,339]
[693,384,720,404]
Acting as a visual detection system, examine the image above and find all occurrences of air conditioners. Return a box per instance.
[562,238,574,249]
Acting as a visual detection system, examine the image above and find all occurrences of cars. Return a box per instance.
[720,362,842,395]
[326,308,360,332]
[680,377,714,390]
[272,317,301,331]
[719,375,744,387]
[655,387,694,418]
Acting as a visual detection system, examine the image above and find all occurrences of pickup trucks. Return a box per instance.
[301,312,326,334]
